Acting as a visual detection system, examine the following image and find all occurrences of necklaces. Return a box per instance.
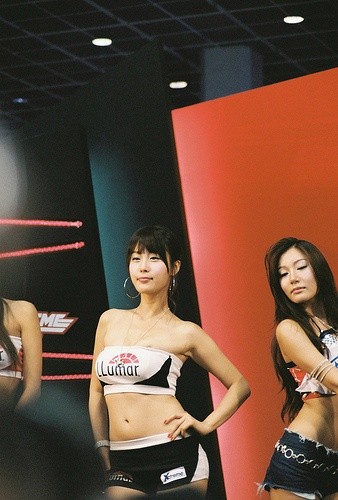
[118,307,170,366]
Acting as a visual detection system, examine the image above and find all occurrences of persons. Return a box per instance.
[89,224,251,500]
[0,295,42,417]
[255,236,338,500]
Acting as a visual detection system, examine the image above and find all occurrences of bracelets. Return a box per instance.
[93,439,110,453]
[309,358,335,384]
